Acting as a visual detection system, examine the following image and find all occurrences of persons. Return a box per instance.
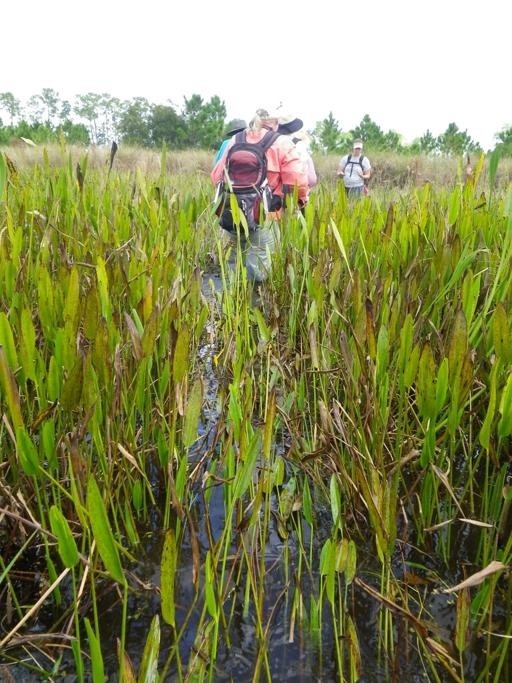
[276,109,317,190]
[210,105,309,291]
[336,138,371,198]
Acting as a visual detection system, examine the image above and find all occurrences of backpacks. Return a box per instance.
[213,129,290,234]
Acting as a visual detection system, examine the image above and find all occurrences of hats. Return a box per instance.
[225,119,248,137]
[353,138,363,150]
[256,101,293,120]
[278,114,303,135]
